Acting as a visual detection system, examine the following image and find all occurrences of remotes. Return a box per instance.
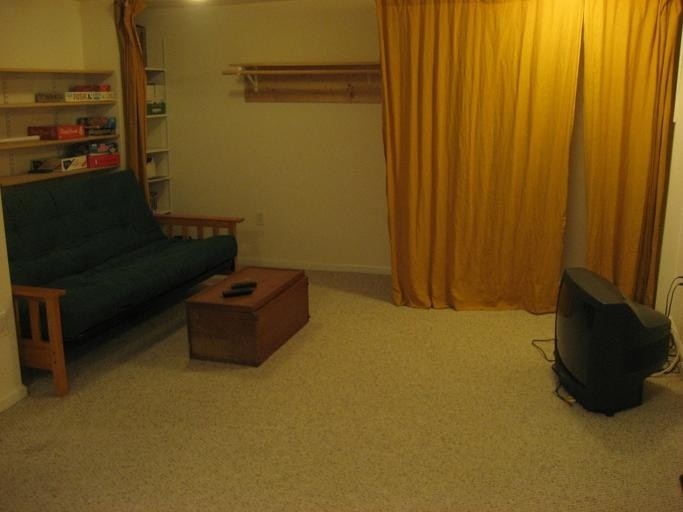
[231,280,257,289]
[223,288,254,298]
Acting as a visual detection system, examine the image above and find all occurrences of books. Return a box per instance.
[35,85,115,102]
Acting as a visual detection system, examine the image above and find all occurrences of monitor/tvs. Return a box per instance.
[552,269,670,417]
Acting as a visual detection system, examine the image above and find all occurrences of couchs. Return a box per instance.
[2,165,244,395]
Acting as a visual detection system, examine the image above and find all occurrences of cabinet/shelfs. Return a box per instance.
[144,67,172,215]
[0,66,121,189]
